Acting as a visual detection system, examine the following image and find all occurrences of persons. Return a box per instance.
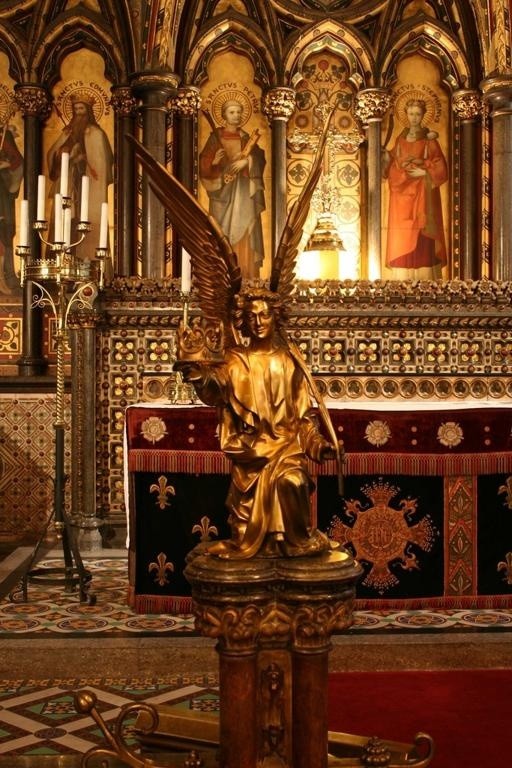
[198,97,267,283]
[183,288,341,556]
[1,120,22,294]
[42,95,113,263]
[379,94,448,281]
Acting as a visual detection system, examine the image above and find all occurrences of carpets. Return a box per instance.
[1,556,512,640]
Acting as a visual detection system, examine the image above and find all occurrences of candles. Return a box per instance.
[16,150,109,252]
